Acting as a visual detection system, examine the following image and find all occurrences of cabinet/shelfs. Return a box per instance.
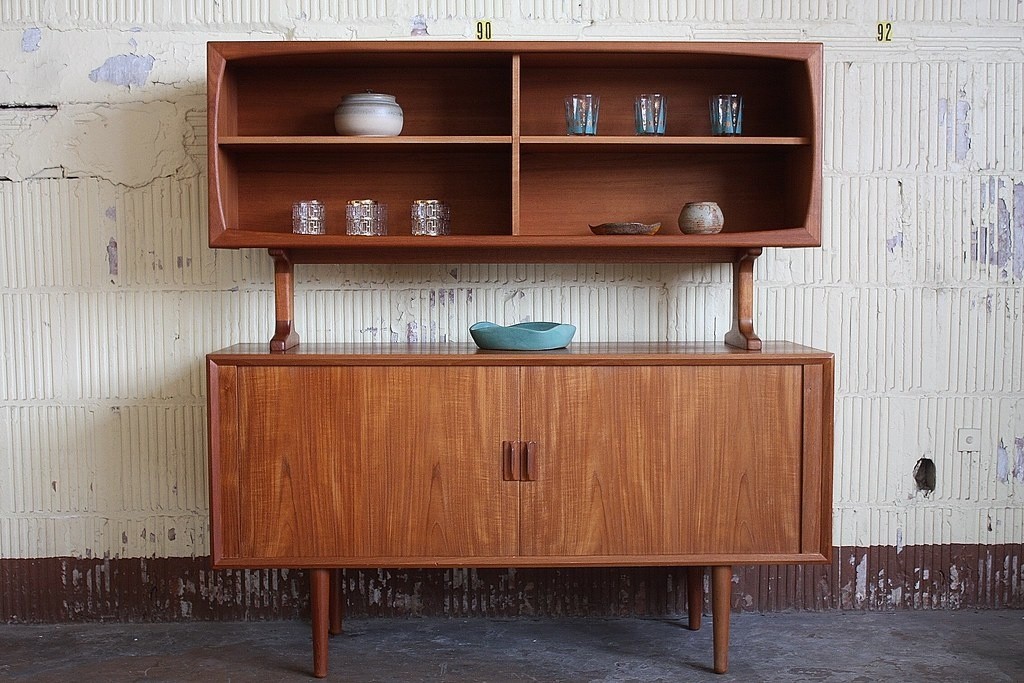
[206,340,833,565]
[207,40,823,249]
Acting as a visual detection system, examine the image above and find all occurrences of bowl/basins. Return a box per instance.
[678,201,724,235]
[335,93,403,136]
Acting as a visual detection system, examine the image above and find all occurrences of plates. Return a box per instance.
[469,322,576,350]
[589,222,661,234]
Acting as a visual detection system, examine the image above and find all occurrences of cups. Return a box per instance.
[633,94,666,136]
[565,94,599,136]
[709,94,743,136]
